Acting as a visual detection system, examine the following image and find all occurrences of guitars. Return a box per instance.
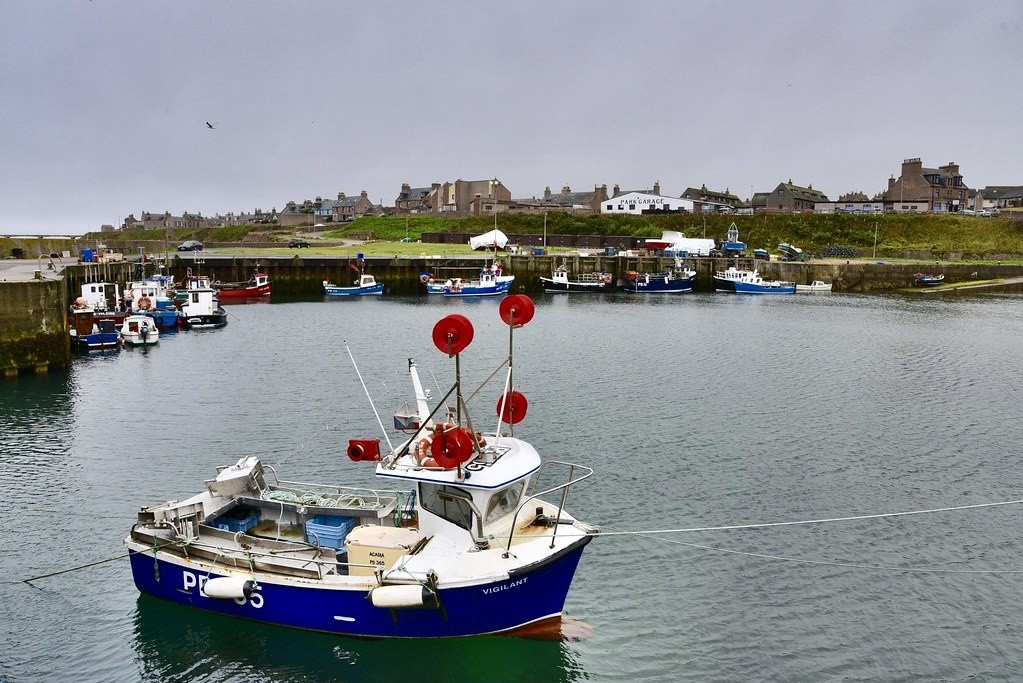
[605,276,611,283]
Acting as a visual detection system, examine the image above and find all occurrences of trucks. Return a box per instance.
[885,206,898,215]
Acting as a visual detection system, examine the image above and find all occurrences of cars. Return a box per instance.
[873,208,882,215]
[288,239,310,249]
[804,209,815,214]
[852,208,863,214]
[837,208,850,214]
[957,207,1000,219]
[177,240,203,251]
[820,208,829,214]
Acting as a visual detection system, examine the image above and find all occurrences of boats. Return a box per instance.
[794,280,833,293]
[913,273,946,288]
[778,243,801,259]
[538,260,615,293]
[644,239,671,251]
[122,293,601,642]
[211,260,273,297]
[722,221,749,254]
[732,275,796,295]
[64,247,227,351]
[322,273,385,295]
[623,254,698,293]
[754,248,770,259]
[418,240,516,297]
[712,262,765,292]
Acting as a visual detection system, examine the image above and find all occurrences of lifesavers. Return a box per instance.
[450,285,457,293]
[138,297,151,309]
[435,423,487,447]
[415,432,440,467]
[420,275,429,282]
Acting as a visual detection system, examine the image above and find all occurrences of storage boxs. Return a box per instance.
[214,509,261,533]
[305,515,356,548]
